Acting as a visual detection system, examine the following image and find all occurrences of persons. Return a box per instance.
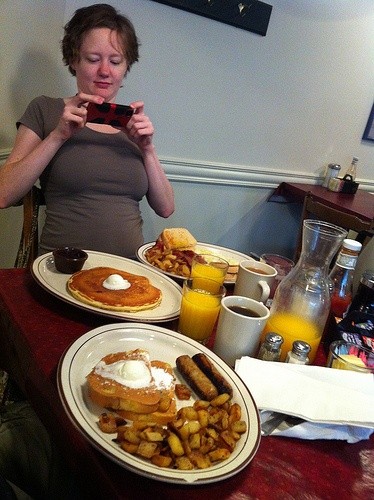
[0,3,175,264]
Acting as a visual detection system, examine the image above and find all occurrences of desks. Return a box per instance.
[279,184,374,270]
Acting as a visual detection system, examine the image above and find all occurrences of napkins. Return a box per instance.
[234,358,374,430]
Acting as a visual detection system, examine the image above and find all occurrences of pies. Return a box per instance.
[68,266,162,313]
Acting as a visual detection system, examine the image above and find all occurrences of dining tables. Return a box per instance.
[0,266,374,500]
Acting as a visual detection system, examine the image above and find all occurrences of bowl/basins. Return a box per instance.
[53,247,88,274]
[326,341,374,374]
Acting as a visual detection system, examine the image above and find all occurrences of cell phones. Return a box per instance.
[86,102,133,128]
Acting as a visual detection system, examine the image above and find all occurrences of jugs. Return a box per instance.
[261,219,347,365]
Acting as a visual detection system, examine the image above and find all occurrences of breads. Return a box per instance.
[224,265,239,282]
[157,228,197,253]
[87,349,178,423]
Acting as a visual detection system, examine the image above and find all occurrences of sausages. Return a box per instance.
[193,353,233,400]
[175,355,218,402]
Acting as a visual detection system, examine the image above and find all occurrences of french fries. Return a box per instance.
[145,249,179,272]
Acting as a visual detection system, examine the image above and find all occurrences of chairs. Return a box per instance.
[12,177,47,268]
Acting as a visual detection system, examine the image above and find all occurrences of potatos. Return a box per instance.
[99,384,247,470]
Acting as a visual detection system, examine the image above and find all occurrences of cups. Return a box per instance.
[190,254,229,284]
[210,295,271,368]
[177,277,226,346]
[259,252,295,300]
[232,260,278,306]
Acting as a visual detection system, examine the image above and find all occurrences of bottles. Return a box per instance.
[325,239,362,324]
[286,339,312,364]
[346,156,359,182]
[322,163,341,191]
[256,332,285,363]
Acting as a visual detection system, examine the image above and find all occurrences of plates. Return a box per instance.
[55,322,261,485]
[135,241,256,284]
[30,248,185,321]
